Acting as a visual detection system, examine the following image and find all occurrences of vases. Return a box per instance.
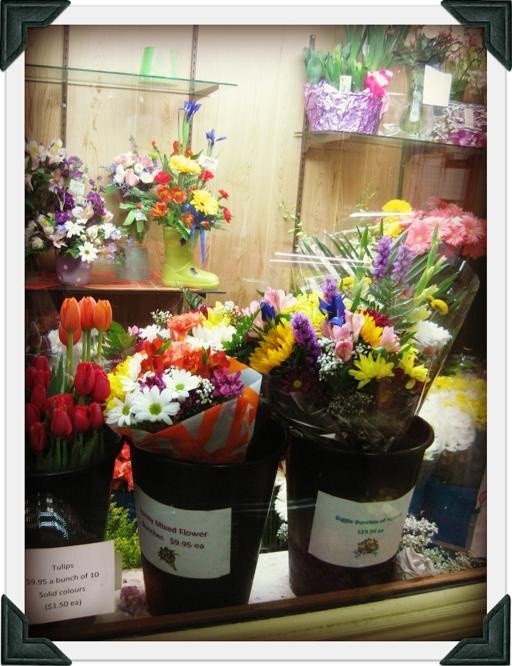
[438,103,487,147]
[305,85,385,135]
[399,64,428,134]
[25,420,433,627]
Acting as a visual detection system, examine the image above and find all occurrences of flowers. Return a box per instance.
[25,96,237,266]
[304,23,487,116]
[22,199,486,485]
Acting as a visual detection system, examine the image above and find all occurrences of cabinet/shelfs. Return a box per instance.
[23,61,239,295]
